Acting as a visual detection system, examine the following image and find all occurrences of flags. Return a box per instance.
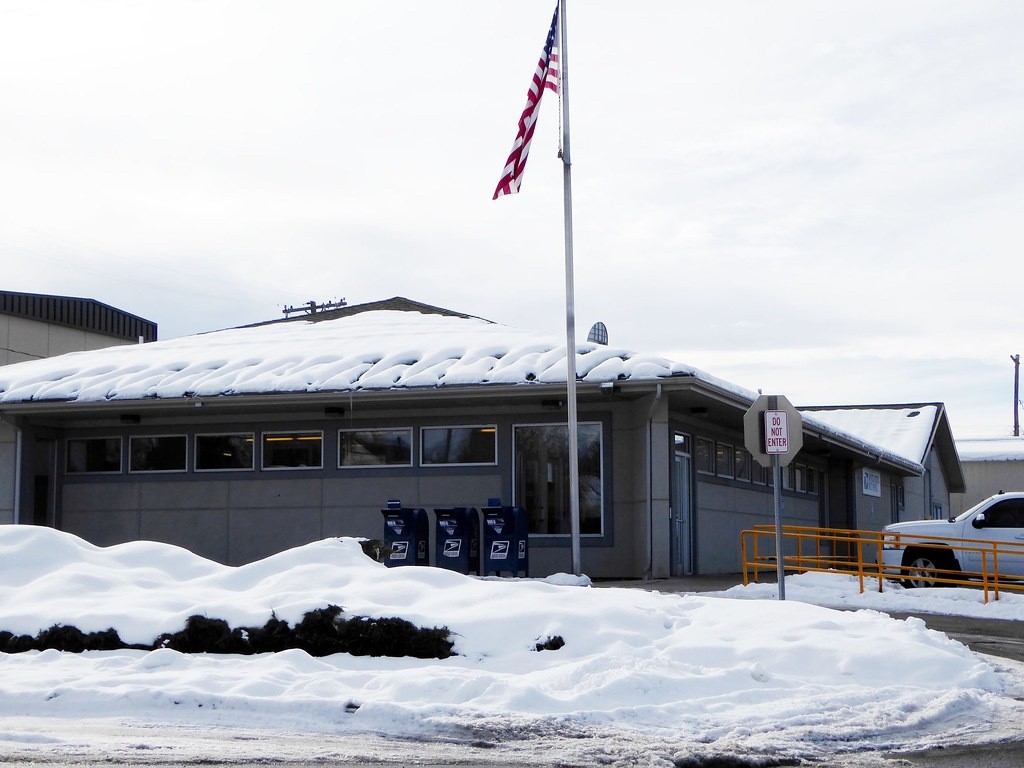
[492,0,563,200]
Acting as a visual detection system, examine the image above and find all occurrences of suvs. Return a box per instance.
[875,489,1024,590]
